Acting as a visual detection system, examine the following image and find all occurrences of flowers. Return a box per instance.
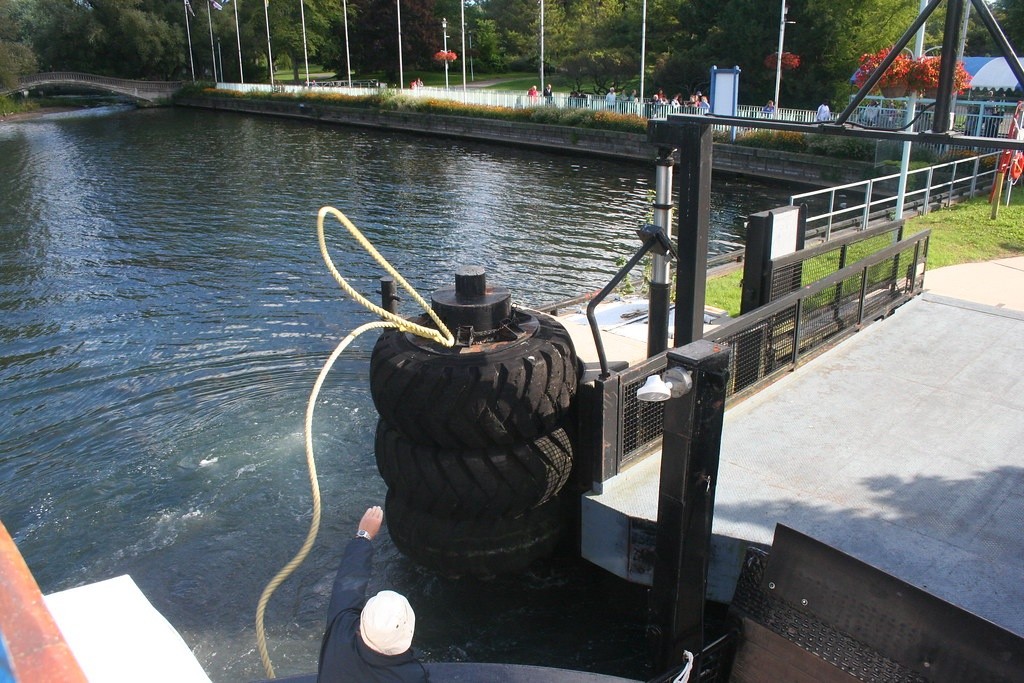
[852,45,973,97]
[764,52,799,70]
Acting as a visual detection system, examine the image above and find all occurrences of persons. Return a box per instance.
[607,87,637,113]
[647,89,710,119]
[528,86,538,105]
[960,92,1006,138]
[763,100,775,119]
[317,505,429,683]
[816,99,832,121]
[544,84,553,106]
[410,78,423,89]
[568,90,589,108]
[857,100,932,132]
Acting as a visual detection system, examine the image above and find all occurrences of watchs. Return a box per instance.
[355,530,372,541]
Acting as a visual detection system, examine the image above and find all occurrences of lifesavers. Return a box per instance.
[385,487,571,582]
[375,415,581,520]
[369,303,578,449]
[1010,152,1024,180]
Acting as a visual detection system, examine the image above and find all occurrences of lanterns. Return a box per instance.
[435,50,446,63]
[446,50,457,62]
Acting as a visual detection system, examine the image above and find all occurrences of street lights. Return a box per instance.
[441,17,452,90]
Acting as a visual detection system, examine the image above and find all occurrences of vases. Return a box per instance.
[879,82,906,97]
[925,87,938,99]
[783,65,792,70]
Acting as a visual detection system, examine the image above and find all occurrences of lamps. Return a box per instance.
[586,226,677,378]
[635,366,692,402]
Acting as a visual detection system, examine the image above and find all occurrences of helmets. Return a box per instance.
[360,590,415,655]
